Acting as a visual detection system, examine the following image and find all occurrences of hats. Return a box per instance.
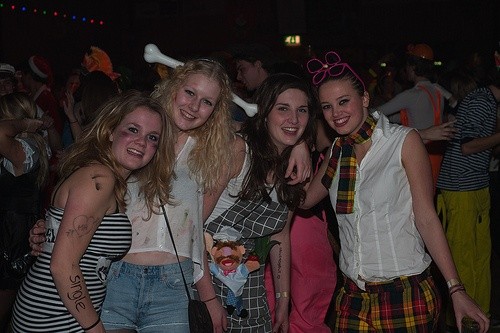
[28,54,54,92]
[0,63,18,77]
[400,42,434,62]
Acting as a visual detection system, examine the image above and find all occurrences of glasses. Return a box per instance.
[307,51,367,92]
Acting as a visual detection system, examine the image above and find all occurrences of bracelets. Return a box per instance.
[81,317,100,331]
[275,292,289,298]
[202,296,217,302]
[446,278,466,295]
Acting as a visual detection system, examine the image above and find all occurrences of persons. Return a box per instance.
[0,28,500,333]
[8,90,175,333]
[29,58,233,333]
[298,49,490,333]
[192,73,317,333]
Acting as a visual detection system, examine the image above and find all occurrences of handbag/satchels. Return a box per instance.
[188,299,214,333]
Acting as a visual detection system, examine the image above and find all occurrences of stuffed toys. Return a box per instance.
[204,226,260,319]
